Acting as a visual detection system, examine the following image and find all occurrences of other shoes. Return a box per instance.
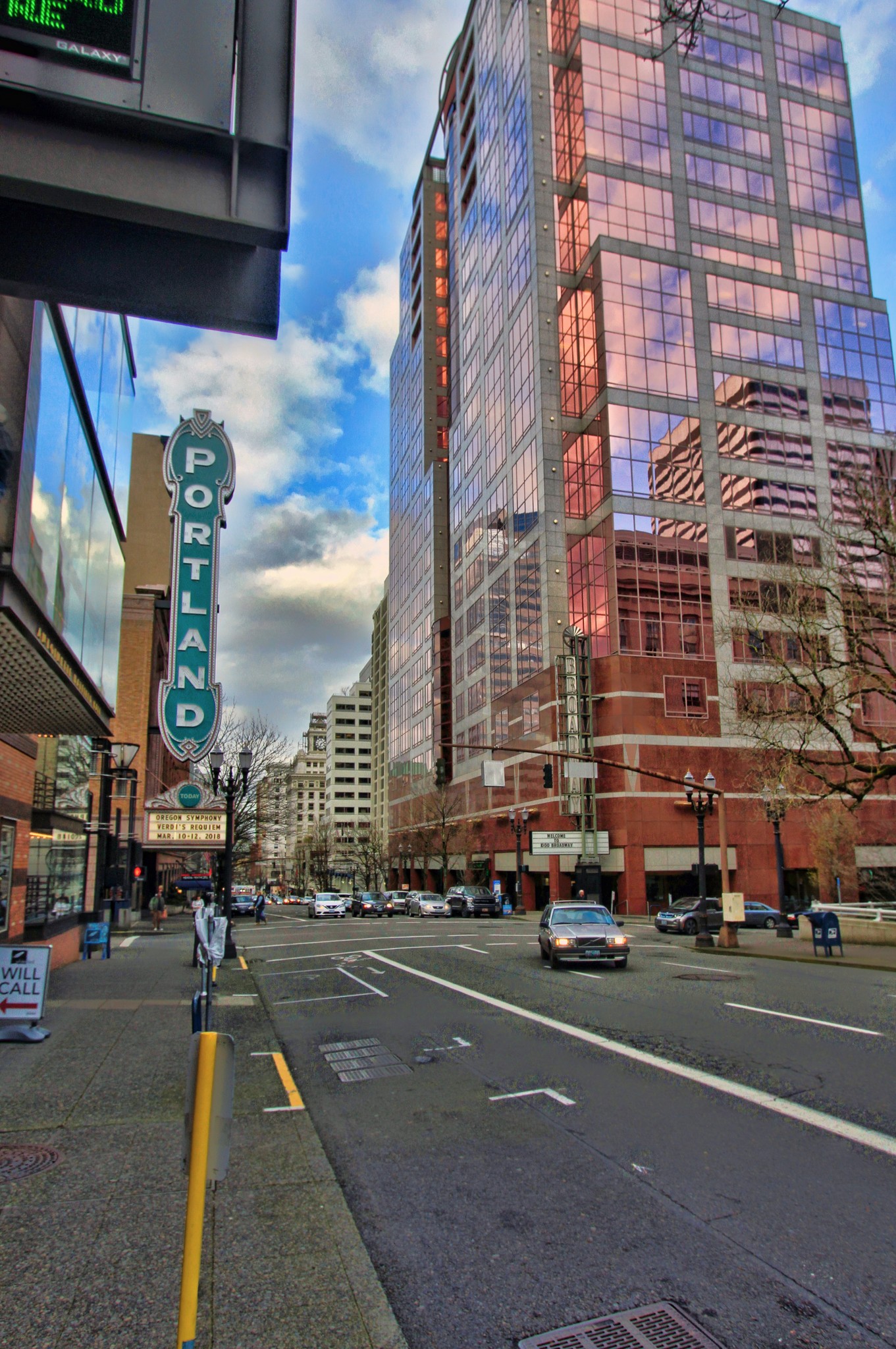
[159,928,163,931]
[154,927,157,931]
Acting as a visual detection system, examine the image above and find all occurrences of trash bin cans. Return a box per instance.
[498,893,513,916]
[803,912,844,958]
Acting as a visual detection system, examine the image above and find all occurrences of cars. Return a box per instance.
[407,893,452,918]
[382,890,409,915]
[248,894,312,906]
[786,909,813,929]
[336,893,353,909]
[536,899,629,969]
[737,901,782,930]
[307,892,346,918]
[351,891,394,918]
[230,895,256,916]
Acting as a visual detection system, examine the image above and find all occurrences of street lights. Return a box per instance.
[680,767,718,949]
[508,806,530,916]
[207,741,254,958]
[761,777,794,939]
[398,843,413,892]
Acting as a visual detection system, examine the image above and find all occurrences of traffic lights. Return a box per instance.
[435,758,447,785]
[543,763,554,788]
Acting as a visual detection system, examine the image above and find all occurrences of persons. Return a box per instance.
[149,891,165,931]
[252,891,268,925]
[191,894,205,925]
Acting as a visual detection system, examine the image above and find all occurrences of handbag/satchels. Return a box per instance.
[161,906,168,918]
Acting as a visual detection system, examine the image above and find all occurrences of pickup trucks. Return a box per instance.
[445,885,500,918]
[654,896,739,934]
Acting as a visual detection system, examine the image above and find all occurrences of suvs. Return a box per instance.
[404,890,434,916]
